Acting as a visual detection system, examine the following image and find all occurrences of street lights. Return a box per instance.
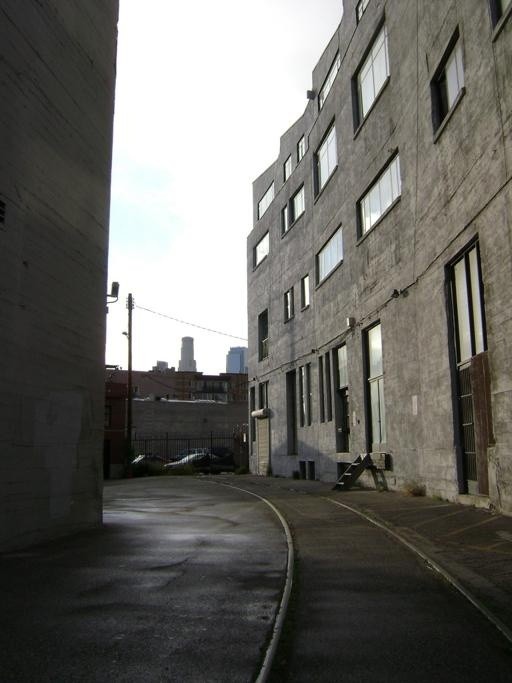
[121,331,133,477]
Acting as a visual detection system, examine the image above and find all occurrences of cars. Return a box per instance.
[127,445,234,476]
